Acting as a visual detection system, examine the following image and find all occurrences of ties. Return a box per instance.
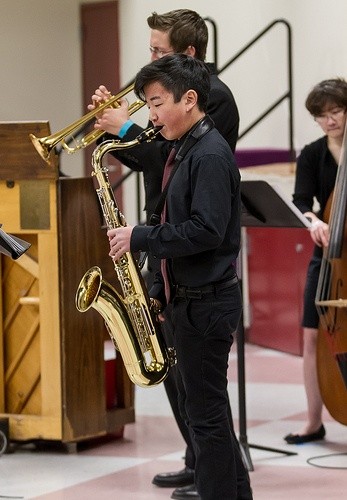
[159,148,174,304]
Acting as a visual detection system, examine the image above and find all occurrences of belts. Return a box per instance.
[170,275,239,300]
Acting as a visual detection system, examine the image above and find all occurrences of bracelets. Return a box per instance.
[117,118,135,138]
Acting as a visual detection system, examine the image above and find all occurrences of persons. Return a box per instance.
[86,10,257,500]
[284,77,347,445]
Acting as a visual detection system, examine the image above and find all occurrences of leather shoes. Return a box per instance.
[170,485,202,500]
[152,465,196,487]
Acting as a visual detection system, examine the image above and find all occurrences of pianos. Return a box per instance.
[0,120,138,455]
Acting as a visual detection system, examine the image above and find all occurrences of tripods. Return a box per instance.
[235,179,310,471]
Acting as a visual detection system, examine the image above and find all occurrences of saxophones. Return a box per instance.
[75,125,178,389]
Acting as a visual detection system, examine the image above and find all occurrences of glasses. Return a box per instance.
[311,104,346,122]
[149,46,175,58]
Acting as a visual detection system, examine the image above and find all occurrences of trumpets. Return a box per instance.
[29,80,147,167]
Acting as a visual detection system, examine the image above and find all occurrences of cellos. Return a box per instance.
[314,116,347,428]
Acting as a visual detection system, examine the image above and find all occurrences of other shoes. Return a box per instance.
[284,423,326,444]
